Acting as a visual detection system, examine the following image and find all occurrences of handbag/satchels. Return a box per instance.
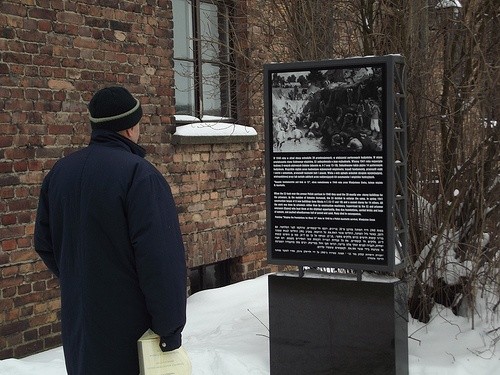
[137,328,192,375]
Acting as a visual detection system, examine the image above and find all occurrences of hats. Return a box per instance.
[87,87,142,132]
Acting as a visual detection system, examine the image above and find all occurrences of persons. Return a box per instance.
[34,86,192,375]
[273,99,381,152]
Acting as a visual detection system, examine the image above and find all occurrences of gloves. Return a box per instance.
[159,333,181,352]
